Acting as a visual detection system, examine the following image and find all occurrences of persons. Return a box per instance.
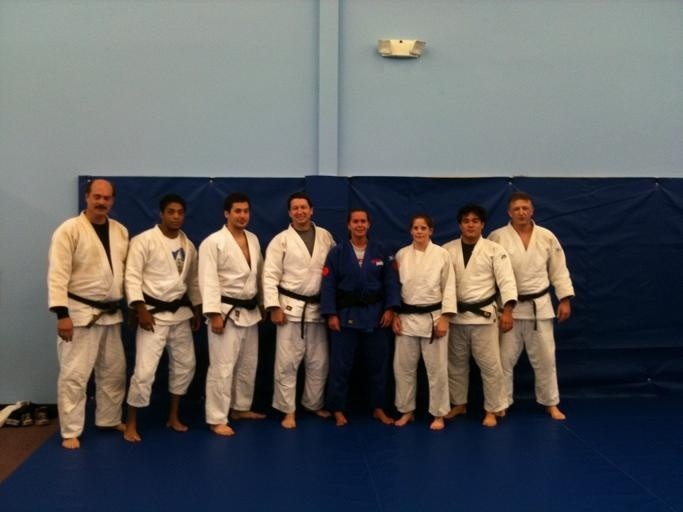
[389,214,458,429]
[485,191,578,421]
[260,192,337,430]
[44,179,131,449]
[317,208,402,428]
[196,191,270,437]
[440,205,519,428]
[119,195,201,441]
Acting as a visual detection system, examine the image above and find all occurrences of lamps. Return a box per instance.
[375,38,426,58]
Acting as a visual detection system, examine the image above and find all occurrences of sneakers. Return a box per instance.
[4,405,51,428]
